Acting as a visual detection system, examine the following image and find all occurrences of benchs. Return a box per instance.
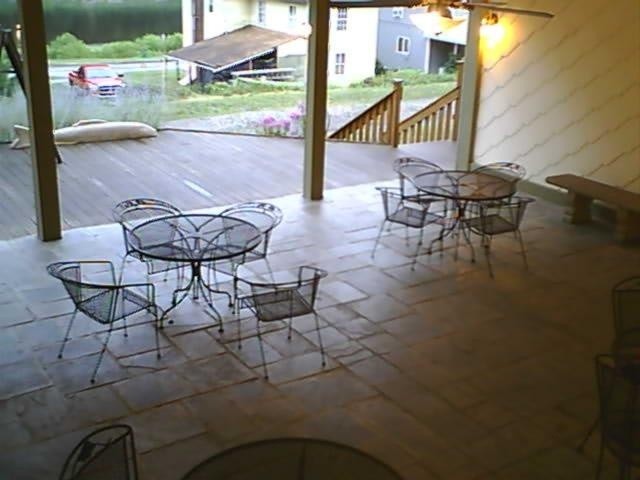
[544,169,640,248]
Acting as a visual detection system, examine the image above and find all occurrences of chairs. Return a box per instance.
[234,265,328,381]
[112,197,200,313]
[45,260,162,384]
[571,272,638,454]
[586,345,640,480]
[56,420,142,480]
[366,150,540,281]
[192,202,283,314]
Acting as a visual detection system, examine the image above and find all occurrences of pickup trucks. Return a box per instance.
[68,63,127,99]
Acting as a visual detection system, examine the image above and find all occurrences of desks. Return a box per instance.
[126,213,262,334]
[178,436,405,479]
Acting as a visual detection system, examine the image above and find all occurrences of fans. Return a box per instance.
[328,0,555,23]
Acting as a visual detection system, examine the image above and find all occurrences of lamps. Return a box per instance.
[408,3,466,38]
